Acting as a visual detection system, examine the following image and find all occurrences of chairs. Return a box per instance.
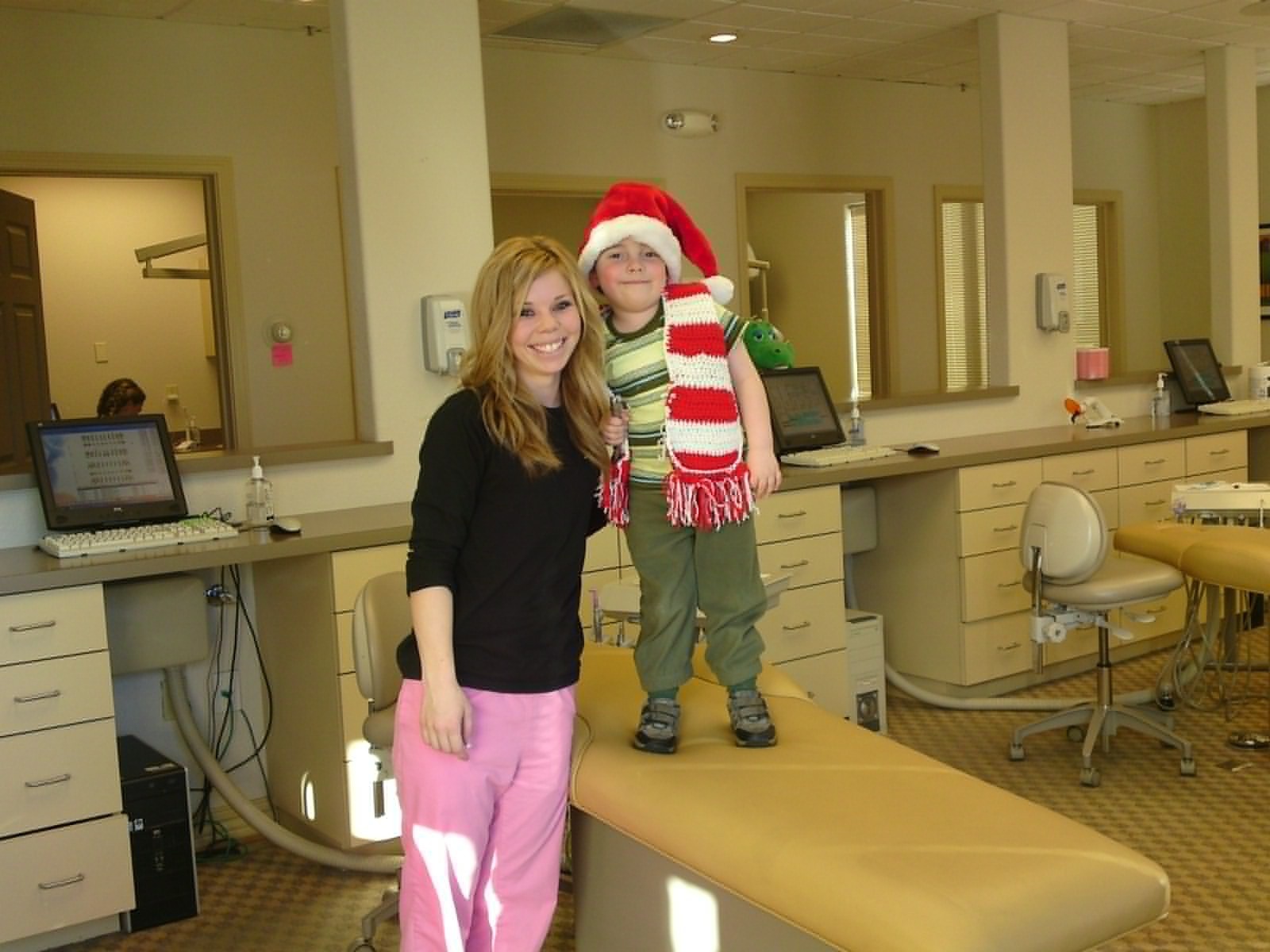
[1002,478,1199,787]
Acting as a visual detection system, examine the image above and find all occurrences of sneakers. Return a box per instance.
[634,703,680,754]
[725,696,777,746]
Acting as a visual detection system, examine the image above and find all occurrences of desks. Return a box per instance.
[712,401,1269,718]
[0,498,452,951]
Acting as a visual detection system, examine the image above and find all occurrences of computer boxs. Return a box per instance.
[846,609,888,736]
[116,735,201,933]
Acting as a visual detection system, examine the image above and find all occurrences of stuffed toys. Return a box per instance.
[743,317,795,371]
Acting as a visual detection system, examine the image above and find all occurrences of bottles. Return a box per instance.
[1152,372,1172,416]
[848,397,865,445]
[244,454,274,529]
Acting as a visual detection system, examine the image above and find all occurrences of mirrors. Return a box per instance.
[933,183,1123,406]
[735,169,895,413]
[1,148,238,452]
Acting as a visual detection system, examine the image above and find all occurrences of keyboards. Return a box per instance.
[38,516,238,558]
[1196,399,1270,415]
[780,443,895,466]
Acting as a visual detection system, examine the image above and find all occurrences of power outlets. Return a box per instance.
[212,669,244,713]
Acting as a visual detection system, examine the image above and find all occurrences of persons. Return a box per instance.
[578,178,782,752]
[98,377,144,418]
[390,233,630,952]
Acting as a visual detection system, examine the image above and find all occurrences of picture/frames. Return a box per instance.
[1255,223,1270,321]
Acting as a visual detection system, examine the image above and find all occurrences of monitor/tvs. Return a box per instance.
[1165,338,1231,403]
[758,365,846,452]
[26,413,186,529]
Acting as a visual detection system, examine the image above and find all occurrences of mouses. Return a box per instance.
[268,517,300,536]
[907,443,940,453]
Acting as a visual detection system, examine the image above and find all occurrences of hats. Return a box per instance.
[578,179,737,307]
